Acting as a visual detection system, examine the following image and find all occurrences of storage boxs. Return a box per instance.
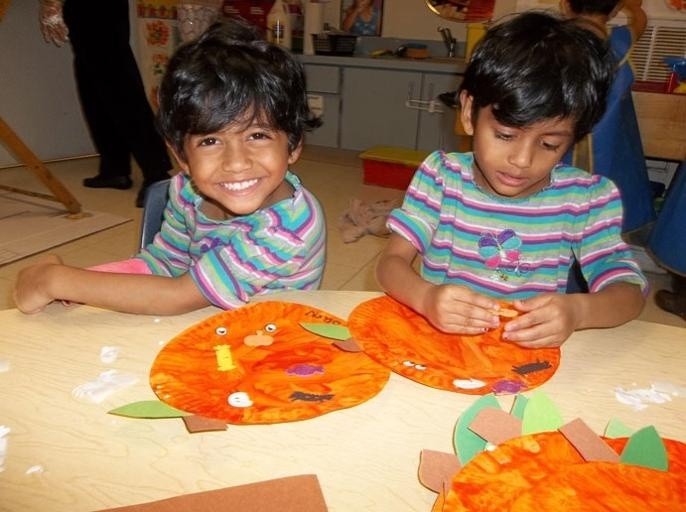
[359,145,426,189]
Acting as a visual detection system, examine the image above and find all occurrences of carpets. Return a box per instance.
[0,184,135,268]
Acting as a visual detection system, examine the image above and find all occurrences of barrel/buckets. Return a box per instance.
[464,21,487,65]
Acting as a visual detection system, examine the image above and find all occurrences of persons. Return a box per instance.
[558,0,659,294]
[14,34,325,316]
[643,161,686,319]
[342,0,378,36]
[376,11,650,349]
[38,1,175,208]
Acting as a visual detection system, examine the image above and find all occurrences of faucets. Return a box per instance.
[439,27,457,57]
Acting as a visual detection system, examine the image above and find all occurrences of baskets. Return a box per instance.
[311,33,357,56]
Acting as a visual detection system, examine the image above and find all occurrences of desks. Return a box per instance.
[0,289,685,512]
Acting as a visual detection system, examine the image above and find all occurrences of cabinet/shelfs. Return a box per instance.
[339,58,467,156]
[630,90,686,162]
[294,56,341,149]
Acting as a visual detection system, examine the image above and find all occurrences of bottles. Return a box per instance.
[465,22,484,57]
[267,0,292,52]
[303,0,324,55]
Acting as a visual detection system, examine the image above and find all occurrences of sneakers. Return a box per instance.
[655,290,686,320]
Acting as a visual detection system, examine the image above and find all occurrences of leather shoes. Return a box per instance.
[136,185,146,207]
[84,174,132,190]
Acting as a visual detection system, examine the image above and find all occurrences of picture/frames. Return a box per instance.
[338,0,386,37]
